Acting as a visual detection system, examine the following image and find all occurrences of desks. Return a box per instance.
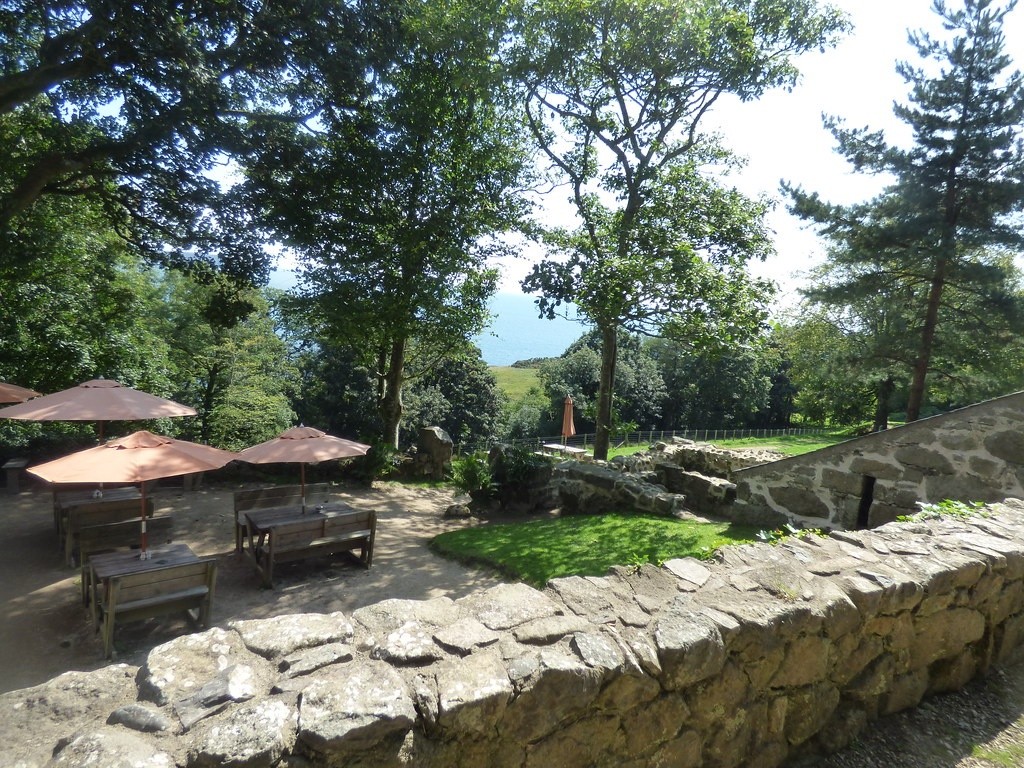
[3,458,32,493]
[543,444,588,460]
[54,487,143,550]
[88,544,200,631]
[244,502,360,579]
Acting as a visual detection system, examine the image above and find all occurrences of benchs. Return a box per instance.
[47,484,218,659]
[234,483,378,587]
[533,452,594,463]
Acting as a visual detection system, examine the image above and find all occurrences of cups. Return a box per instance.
[141,552,145,560]
[147,551,151,559]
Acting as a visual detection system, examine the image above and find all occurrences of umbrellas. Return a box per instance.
[0,381,40,404]
[235,426,372,514]
[0,374,197,489]
[26,431,241,561]
[562,394,577,448]
